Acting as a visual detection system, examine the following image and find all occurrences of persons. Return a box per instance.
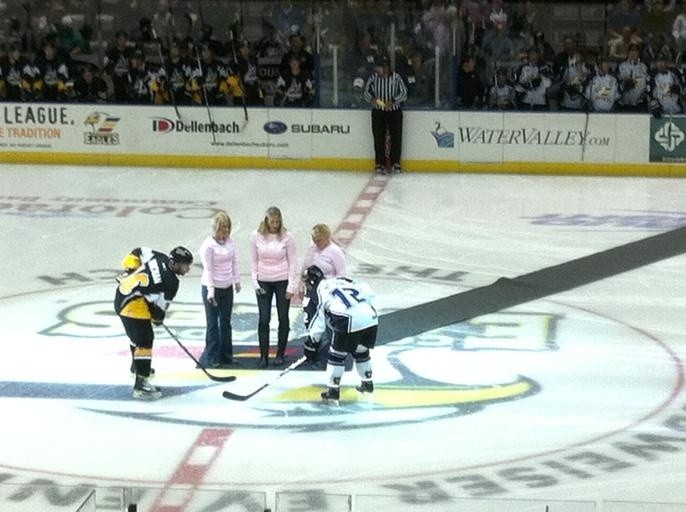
[112,244,196,401]
[301,223,348,362]
[301,263,379,402]
[363,55,409,176]
[198,211,240,368]
[1,1,686,116]
[249,206,297,369]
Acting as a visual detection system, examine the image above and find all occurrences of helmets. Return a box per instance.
[301,265,323,291]
[169,246,193,264]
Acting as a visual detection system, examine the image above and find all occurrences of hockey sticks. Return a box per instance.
[225,30,248,121]
[151,14,180,119]
[191,30,215,145]
[223,339,331,401]
[162,324,236,382]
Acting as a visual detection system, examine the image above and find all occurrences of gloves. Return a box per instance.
[303,335,318,361]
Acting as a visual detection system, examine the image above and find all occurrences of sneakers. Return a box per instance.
[321,387,339,399]
[356,380,373,392]
[130,358,154,374]
[134,376,160,391]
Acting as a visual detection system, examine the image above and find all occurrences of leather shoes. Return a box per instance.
[274,356,284,365]
[231,358,239,364]
[259,355,267,368]
[209,360,221,368]
